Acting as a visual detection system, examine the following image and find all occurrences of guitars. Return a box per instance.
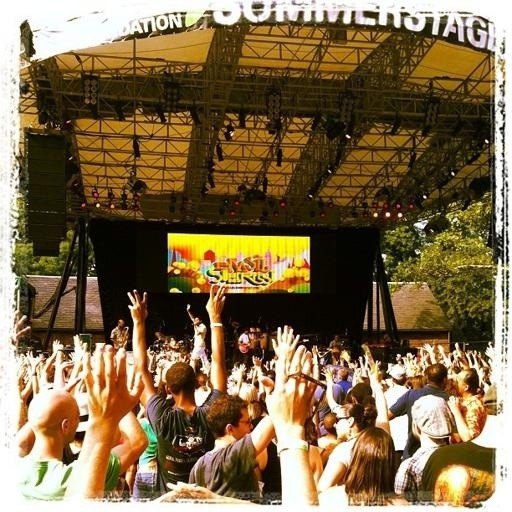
[239,338,264,354]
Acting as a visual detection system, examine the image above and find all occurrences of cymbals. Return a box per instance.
[339,338,348,342]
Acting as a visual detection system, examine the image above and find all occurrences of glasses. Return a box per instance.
[289,369,328,424]
[239,416,253,428]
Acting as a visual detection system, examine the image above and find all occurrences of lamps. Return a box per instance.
[18,66,495,225]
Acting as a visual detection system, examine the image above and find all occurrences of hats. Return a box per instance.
[404,353,416,360]
[410,394,452,440]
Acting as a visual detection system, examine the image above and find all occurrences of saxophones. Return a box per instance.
[118,327,129,348]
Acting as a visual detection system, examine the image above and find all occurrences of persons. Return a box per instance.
[14,285,498,510]
